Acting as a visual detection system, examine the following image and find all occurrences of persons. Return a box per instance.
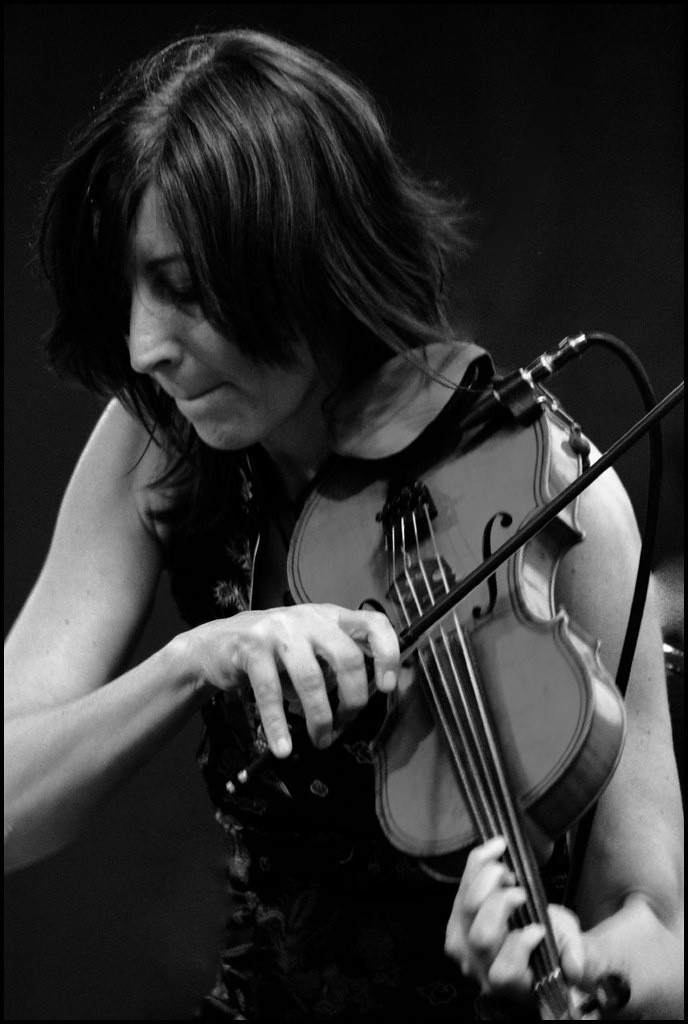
[0,31,688,1023]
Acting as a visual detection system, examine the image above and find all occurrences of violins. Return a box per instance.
[280,365,631,1024]
